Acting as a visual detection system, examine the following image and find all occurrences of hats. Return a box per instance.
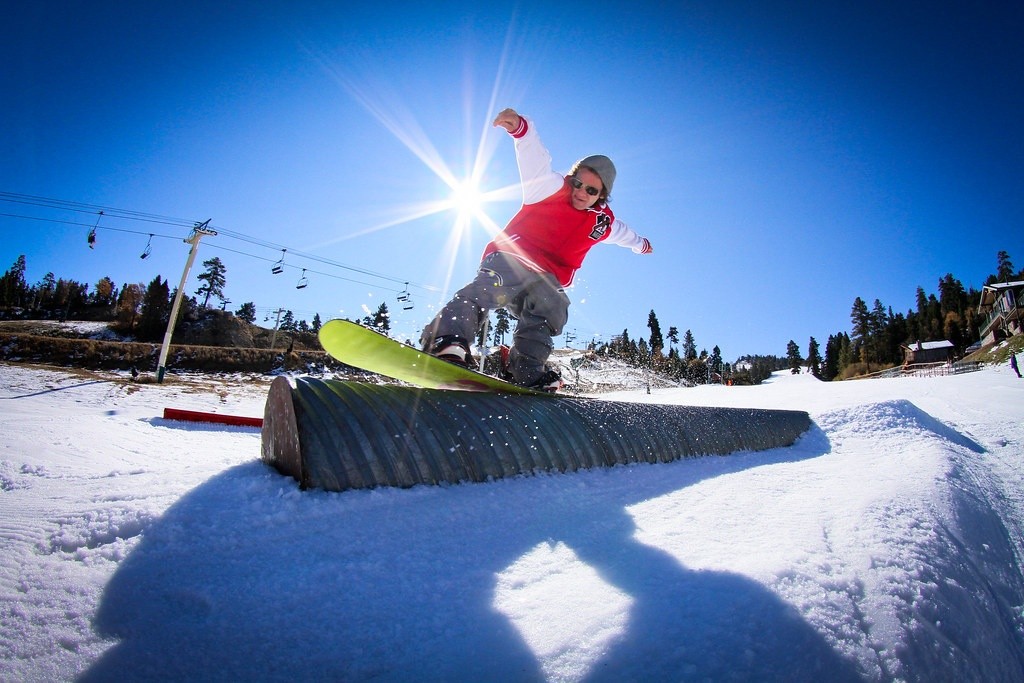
[573,154,617,196]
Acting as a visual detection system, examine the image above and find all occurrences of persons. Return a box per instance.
[1010,351,1024,379]
[419,108,652,392]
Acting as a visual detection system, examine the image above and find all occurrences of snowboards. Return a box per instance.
[316,320,601,401]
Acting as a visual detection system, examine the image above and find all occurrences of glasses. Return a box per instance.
[570,177,600,197]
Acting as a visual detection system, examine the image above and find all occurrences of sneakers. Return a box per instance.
[423,334,469,363]
[530,370,562,392]
